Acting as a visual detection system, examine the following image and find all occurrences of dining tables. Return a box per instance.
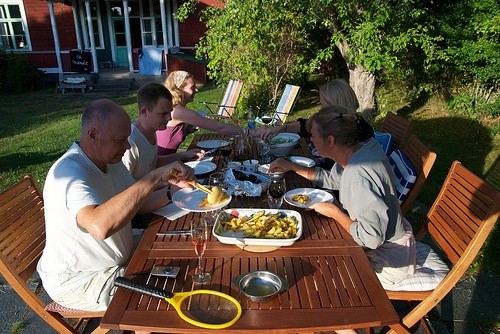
[99,130,400,334]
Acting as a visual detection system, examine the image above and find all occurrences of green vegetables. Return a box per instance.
[271,137,292,144]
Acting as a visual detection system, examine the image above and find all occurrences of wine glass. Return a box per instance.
[270,176,285,208]
[208,173,229,218]
[190,218,211,284]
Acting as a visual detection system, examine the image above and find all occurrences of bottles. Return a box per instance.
[263,141,271,163]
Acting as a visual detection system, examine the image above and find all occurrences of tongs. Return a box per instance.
[169,168,212,194]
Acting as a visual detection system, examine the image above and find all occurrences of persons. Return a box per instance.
[35,98,197,314]
[122,70,417,334]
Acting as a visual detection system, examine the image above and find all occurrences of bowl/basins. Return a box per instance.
[233,270,290,302]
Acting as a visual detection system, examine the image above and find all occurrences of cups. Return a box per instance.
[243,159,259,173]
[228,161,242,171]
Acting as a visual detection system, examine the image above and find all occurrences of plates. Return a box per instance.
[184,161,217,175]
[257,163,285,174]
[269,133,301,147]
[172,185,231,212]
[212,208,301,246]
[197,140,228,149]
[284,188,335,208]
[287,157,315,167]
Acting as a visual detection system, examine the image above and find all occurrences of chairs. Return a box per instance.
[197,78,244,125]
[250,84,301,126]
[363,110,500,334]
[0,175,136,334]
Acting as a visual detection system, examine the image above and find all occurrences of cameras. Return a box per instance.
[151,266,181,279]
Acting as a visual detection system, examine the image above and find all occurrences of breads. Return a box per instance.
[207,186,224,205]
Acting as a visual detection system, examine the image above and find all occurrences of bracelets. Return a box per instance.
[167,185,172,201]
[283,123,288,132]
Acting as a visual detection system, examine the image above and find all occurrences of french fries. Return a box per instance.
[297,194,311,204]
[218,211,298,239]
